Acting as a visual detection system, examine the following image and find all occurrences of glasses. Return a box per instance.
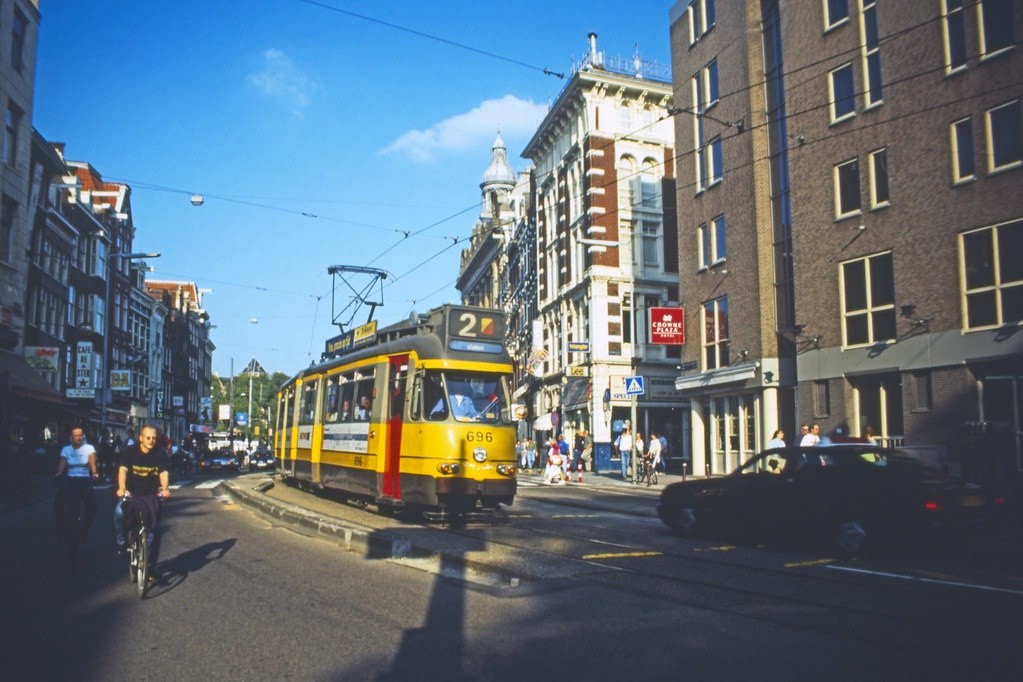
[141,435,156,440]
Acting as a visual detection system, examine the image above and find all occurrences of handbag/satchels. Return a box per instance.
[768,459,778,470]
[552,455,563,465]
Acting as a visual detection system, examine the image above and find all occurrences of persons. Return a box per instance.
[516,431,592,486]
[801,423,825,467]
[656,432,667,471]
[643,433,661,475]
[636,433,644,455]
[115,424,170,583]
[860,426,881,464]
[429,378,482,420]
[49,426,100,542]
[767,430,786,474]
[329,394,371,422]
[613,424,632,480]
[83,428,200,480]
[794,425,808,466]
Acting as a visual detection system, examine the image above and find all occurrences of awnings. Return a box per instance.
[0,351,70,407]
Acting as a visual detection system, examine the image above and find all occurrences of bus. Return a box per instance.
[274,306,519,525]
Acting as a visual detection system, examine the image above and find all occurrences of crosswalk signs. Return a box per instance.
[625,375,644,396]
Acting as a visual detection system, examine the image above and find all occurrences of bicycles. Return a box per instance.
[56,475,97,553]
[182,452,193,480]
[118,492,164,601]
[636,452,658,485]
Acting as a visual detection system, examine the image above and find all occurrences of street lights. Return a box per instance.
[101,251,161,427]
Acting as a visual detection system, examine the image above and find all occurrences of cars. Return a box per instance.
[655,443,1011,559]
[202,450,241,473]
[249,450,277,472]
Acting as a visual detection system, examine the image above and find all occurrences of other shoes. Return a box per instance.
[146,562,159,582]
[114,544,126,556]
